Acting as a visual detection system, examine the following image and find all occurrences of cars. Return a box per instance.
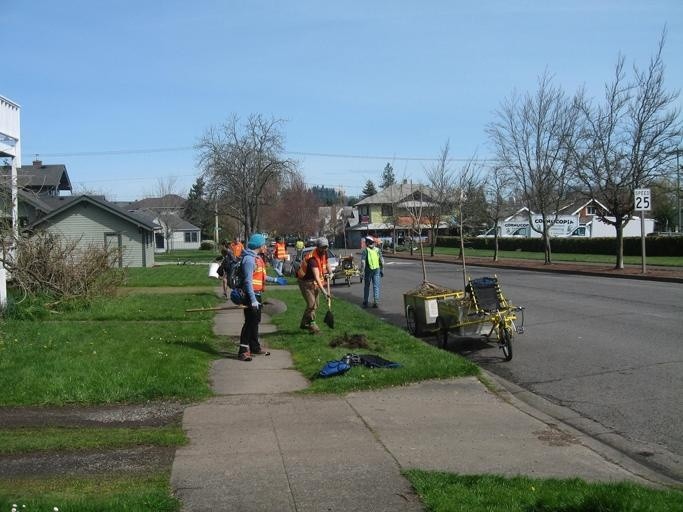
[270,241,276,246]
[287,238,295,246]
[360,229,429,249]
[294,246,339,281]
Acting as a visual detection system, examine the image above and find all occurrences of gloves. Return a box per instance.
[251,301,261,310]
[275,277,288,285]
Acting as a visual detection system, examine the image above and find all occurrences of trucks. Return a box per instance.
[476,214,654,237]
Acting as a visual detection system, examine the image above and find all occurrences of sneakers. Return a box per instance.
[362,301,378,308]
[278,275,286,277]
[299,319,310,329]
[249,349,271,356]
[308,324,325,337]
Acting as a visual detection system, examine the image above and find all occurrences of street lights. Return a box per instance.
[667,148,683,232]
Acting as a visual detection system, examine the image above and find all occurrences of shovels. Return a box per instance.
[324,273,334,329]
[186,298,287,314]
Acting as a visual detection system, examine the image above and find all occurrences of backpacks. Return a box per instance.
[226,253,255,291]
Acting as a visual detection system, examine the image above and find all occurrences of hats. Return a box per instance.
[275,237,282,240]
[315,237,329,248]
[365,235,374,244]
[246,234,265,250]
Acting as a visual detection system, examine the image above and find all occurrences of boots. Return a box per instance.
[237,351,252,361]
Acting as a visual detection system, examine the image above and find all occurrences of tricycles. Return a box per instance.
[334,255,363,287]
[403,274,525,360]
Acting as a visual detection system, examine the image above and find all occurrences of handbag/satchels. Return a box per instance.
[230,288,247,305]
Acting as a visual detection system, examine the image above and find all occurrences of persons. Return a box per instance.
[229,235,246,258]
[216,237,238,301]
[237,233,269,363]
[358,235,385,309]
[293,238,305,262]
[271,235,286,276]
[294,237,334,334]
